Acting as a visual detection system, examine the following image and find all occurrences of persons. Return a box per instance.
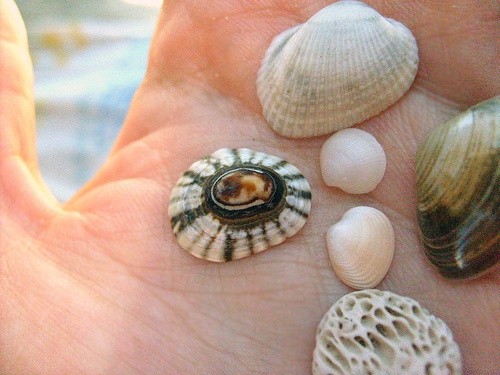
[0,0,500,375]
